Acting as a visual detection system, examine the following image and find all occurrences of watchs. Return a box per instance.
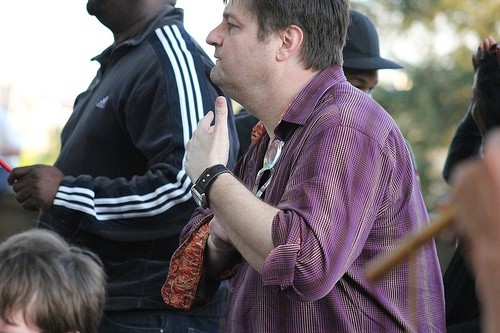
[190,164,231,210]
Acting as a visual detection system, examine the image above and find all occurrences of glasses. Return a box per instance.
[253,139,284,197]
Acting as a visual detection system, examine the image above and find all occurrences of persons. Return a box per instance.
[160,0,446,333]
[443,38,500,333]
[231,10,403,162]
[8,1,241,333]
[0,229,105,333]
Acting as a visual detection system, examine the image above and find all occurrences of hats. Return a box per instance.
[343,10,404,71]
